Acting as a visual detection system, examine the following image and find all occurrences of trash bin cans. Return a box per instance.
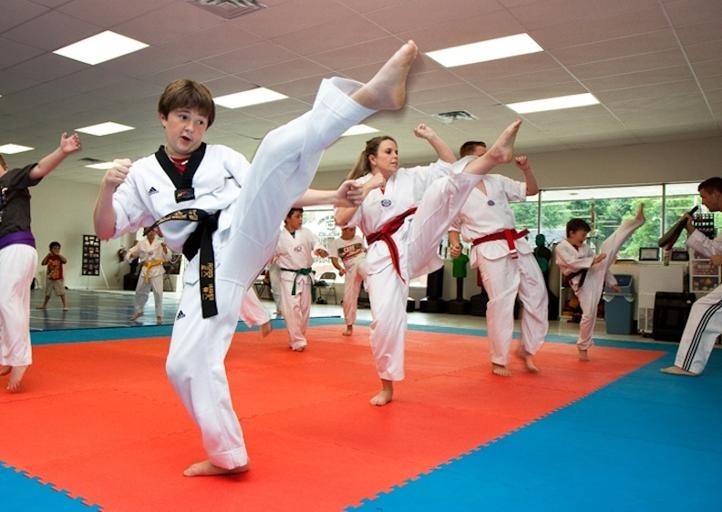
[603,273,635,335]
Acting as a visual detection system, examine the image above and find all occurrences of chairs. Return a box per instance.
[252,269,271,299]
[162,265,173,292]
[314,273,337,304]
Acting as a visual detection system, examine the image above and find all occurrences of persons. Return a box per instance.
[88,38,422,478]
[659,177,722,375]
[333,116,525,411]
[447,140,550,379]
[0,129,82,393]
[40,238,67,307]
[553,201,648,363]
[272,207,330,353]
[126,225,174,325]
[531,233,553,275]
[451,252,470,280]
[330,225,369,338]
[237,285,274,339]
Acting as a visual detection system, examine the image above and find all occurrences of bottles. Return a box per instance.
[663,249,669,266]
[691,212,714,240]
[691,261,718,290]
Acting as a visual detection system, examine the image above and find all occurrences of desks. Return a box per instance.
[608,262,687,336]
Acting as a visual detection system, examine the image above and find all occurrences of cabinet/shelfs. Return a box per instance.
[688,247,722,300]
[558,268,610,324]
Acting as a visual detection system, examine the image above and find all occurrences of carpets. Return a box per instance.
[0,324,667,512]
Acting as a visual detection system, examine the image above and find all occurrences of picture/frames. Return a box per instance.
[671,251,689,261]
[639,247,659,261]
